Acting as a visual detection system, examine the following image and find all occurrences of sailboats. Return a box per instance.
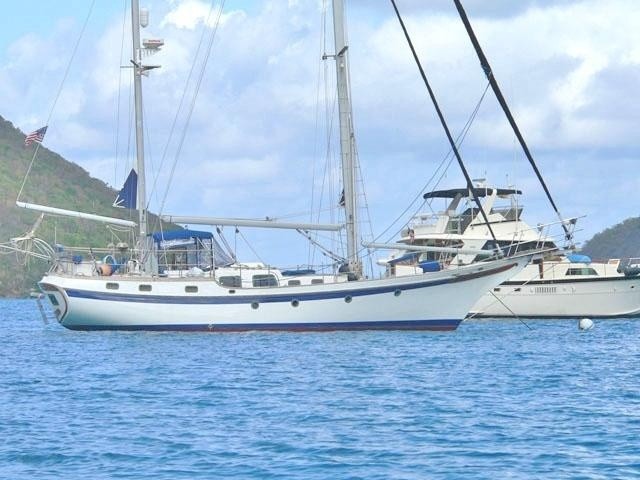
[16,1,587,334]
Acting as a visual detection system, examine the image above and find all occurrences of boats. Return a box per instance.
[378,175,640,321]
